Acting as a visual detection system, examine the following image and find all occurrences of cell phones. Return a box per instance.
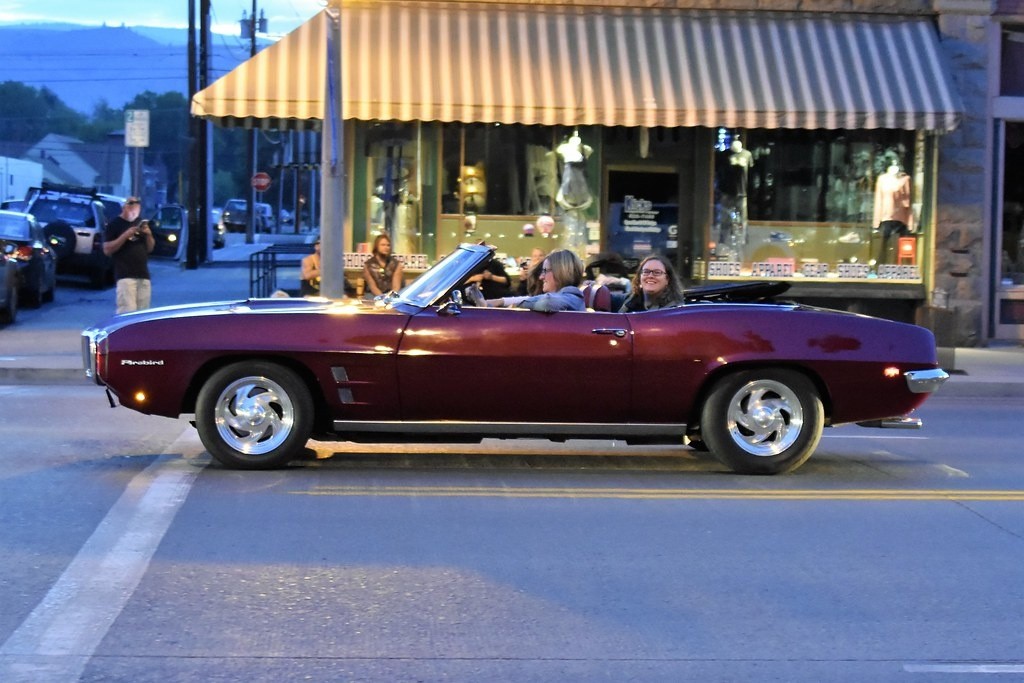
[136,219,149,229]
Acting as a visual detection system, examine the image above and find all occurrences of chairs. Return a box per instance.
[582,285,611,314]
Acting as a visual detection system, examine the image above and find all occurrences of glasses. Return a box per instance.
[639,269,667,276]
[125,201,141,205]
[541,267,553,275]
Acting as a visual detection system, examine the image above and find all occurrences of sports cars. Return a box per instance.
[78,241,950,476]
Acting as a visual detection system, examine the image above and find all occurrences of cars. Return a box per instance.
[0,177,294,326]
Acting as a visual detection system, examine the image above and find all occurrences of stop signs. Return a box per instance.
[251,172,272,192]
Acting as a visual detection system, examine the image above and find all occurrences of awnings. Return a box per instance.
[188,13,966,131]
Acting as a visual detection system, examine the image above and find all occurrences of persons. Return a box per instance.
[459,243,685,315]
[102,196,155,315]
[299,236,358,299]
[554,135,596,211]
[363,234,406,301]
[715,139,754,247]
[871,158,912,272]
